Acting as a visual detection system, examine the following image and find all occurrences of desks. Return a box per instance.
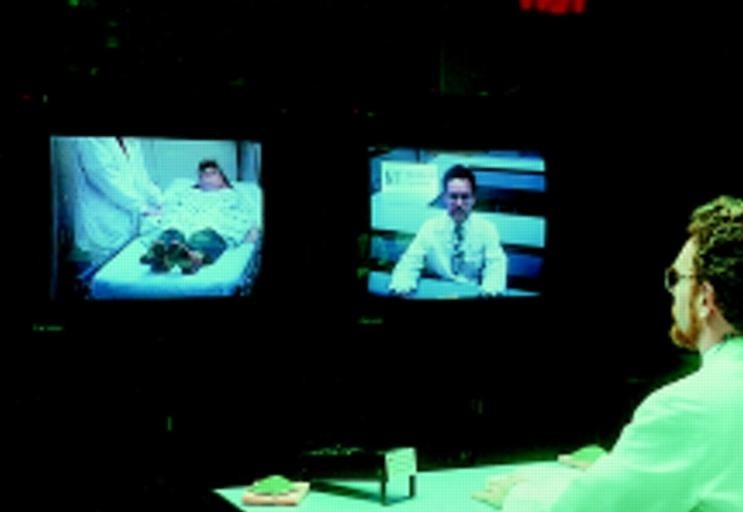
[208,451,587,512]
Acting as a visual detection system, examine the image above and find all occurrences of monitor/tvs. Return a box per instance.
[47,134,267,302]
[368,146,548,305]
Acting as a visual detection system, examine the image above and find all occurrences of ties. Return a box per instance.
[453,227,463,276]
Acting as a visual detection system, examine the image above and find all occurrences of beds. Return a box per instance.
[76,178,263,300]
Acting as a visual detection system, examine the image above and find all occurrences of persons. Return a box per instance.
[141,158,255,274]
[388,165,508,297]
[471,195,743,512]
[68,135,175,278]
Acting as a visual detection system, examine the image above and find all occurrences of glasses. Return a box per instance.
[664,270,697,289]
